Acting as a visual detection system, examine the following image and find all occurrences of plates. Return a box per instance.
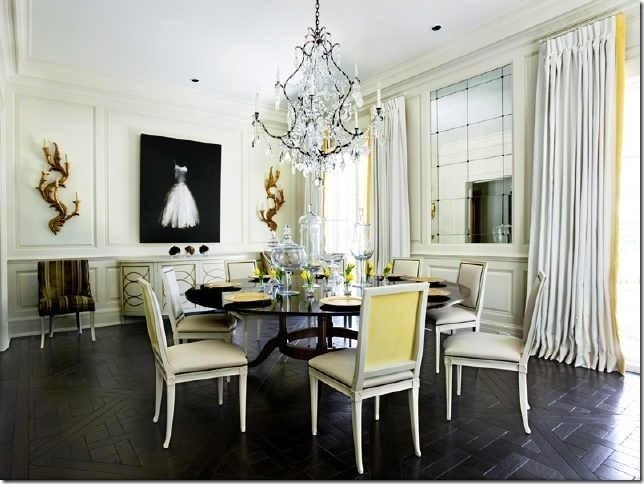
[429,290,450,297]
[318,296,361,307]
[388,273,409,278]
[224,291,270,303]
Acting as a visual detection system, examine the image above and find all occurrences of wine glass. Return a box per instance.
[350,223,375,296]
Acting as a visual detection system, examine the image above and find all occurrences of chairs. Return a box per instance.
[38,260,97,347]
[445,271,546,434]
[162,267,238,383]
[425,263,487,372]
[138,277,251,448]
[390,258,423,278]
[308,282,430,472]
[225,261,261,340]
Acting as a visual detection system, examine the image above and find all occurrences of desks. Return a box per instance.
[185,276,469,367]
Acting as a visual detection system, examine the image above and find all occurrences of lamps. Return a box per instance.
[250,2,389,183]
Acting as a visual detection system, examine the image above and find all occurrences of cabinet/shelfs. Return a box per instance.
[115,258,219,323]
